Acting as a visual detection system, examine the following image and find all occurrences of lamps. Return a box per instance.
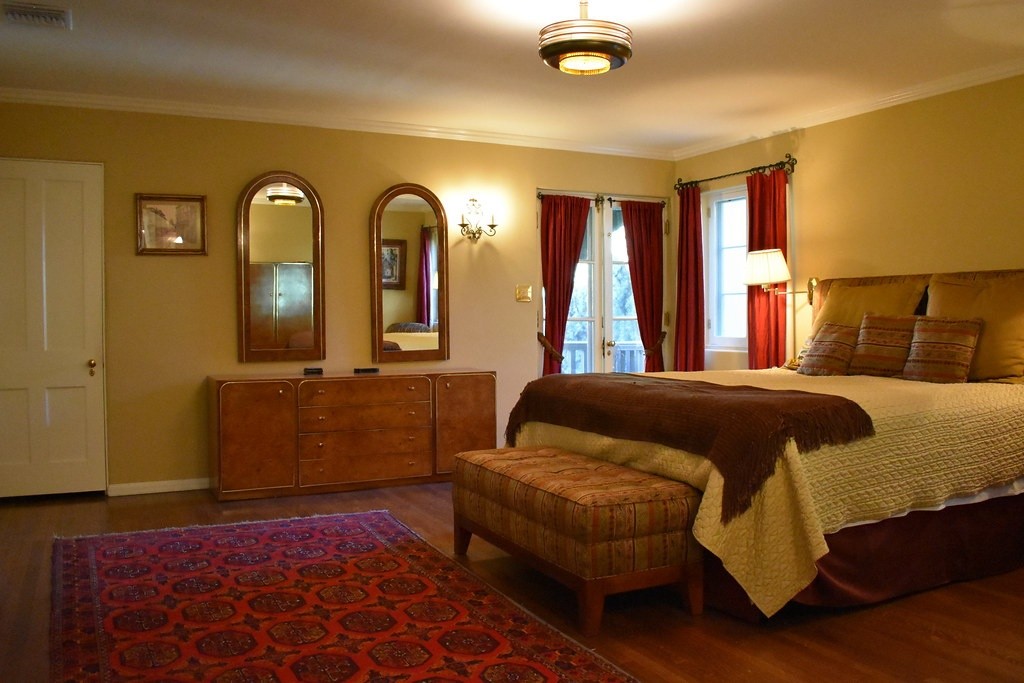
[740,249,818,304]
[457,196,497,242]
[267,184,305,206]
[538,0,633,76]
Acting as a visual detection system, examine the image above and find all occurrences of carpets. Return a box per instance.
[47,508,643,683]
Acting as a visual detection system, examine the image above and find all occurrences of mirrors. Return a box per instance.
[236,171,326,363]
[369,182,450,362]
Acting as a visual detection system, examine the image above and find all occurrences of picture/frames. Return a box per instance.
[381,238,407,289]
[136,192,208,255]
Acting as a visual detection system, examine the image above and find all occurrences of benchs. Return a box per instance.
[452,445,704,638]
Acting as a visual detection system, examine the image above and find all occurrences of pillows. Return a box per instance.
[926,272,1024,384]
[848,311,919,375]
[387,323,430,333]
[785,278,928,370]
[796,321,860,376]
[893,315,982,383]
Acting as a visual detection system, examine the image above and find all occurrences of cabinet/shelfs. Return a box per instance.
[208,369,496,502]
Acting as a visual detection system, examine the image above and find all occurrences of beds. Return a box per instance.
[506,268,1024,617]
